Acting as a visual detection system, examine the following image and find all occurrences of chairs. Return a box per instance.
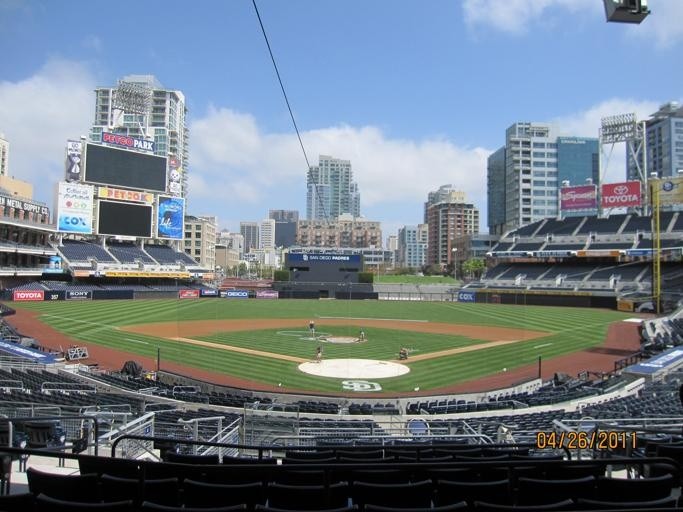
[0,317,683,512]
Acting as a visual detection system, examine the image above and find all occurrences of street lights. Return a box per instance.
[604,0,649,23]
[451,248,458,281]
[593,113,648,216]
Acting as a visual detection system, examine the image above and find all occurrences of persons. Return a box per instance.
[309,320,315,338]
[395,344,408,360]
[360,328,364,341]
[316,344,323,362]
